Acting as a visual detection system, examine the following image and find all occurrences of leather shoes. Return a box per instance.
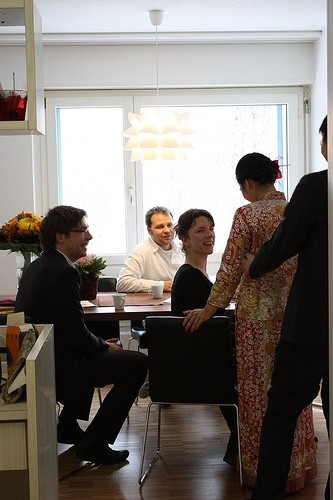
[57,420,85,444]
[68,226,90,235]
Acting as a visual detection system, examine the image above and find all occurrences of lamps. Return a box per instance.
[124,10,192,165]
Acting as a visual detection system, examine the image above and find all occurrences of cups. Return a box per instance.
[112,293,127,310]
[151,286,164,298]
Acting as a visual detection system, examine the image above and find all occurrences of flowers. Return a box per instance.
[74,254,107,276]
[0,210,45,259]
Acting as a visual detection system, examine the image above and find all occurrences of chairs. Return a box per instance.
[84,278,130,423]
[139,314,242,488]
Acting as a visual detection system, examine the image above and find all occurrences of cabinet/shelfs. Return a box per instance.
[0,323,58,500]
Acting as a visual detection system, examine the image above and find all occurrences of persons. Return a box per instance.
[13,205,149,466]
[115,206,186,329]
[241,115,330,500]
[183,152,318,495]
[171,208,238,469]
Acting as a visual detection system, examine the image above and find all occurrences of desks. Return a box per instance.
[0,288,235,321]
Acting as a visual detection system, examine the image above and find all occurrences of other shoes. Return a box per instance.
[76,441,129,464]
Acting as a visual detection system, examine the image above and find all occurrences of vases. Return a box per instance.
[17,252,33,275]
[79,275,100,301]
